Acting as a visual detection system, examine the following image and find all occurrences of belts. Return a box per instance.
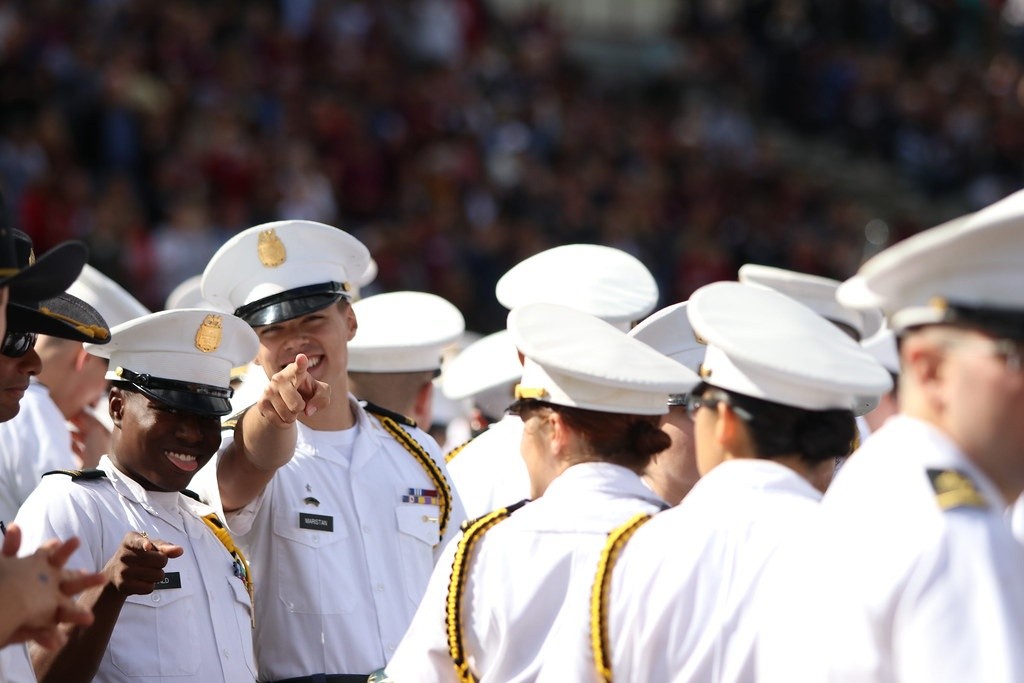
[260,667,389,683]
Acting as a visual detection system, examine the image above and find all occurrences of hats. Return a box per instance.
[166,275,216,310]
[440,330,524,421]
[81,308,260,416]
[627,301,707,405]
[493,243,659,335]
[687,280,893,415]
[64,263,152,329]
[0,227,111,346]
[345,291,466,377]
[503,302,701,418]
[834,269,902,376]
[200,219,371,325]
[739,265,884,342]
[863,189,1024,341]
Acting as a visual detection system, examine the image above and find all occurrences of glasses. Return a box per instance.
[0,332,38,358]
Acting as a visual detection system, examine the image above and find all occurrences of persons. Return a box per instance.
[0,0,1024,683]
